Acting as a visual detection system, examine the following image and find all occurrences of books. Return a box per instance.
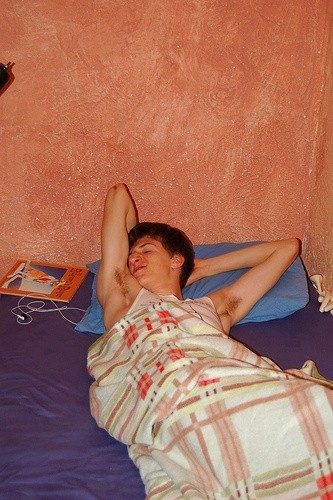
[0,259,89,302]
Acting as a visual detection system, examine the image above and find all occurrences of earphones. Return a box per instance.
[16,313,27,320]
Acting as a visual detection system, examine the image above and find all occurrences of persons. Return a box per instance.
[95,183,299,336]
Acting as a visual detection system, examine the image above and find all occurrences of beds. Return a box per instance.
[1,267,331,500]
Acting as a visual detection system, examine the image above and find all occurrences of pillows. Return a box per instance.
[73,240,311,333]
